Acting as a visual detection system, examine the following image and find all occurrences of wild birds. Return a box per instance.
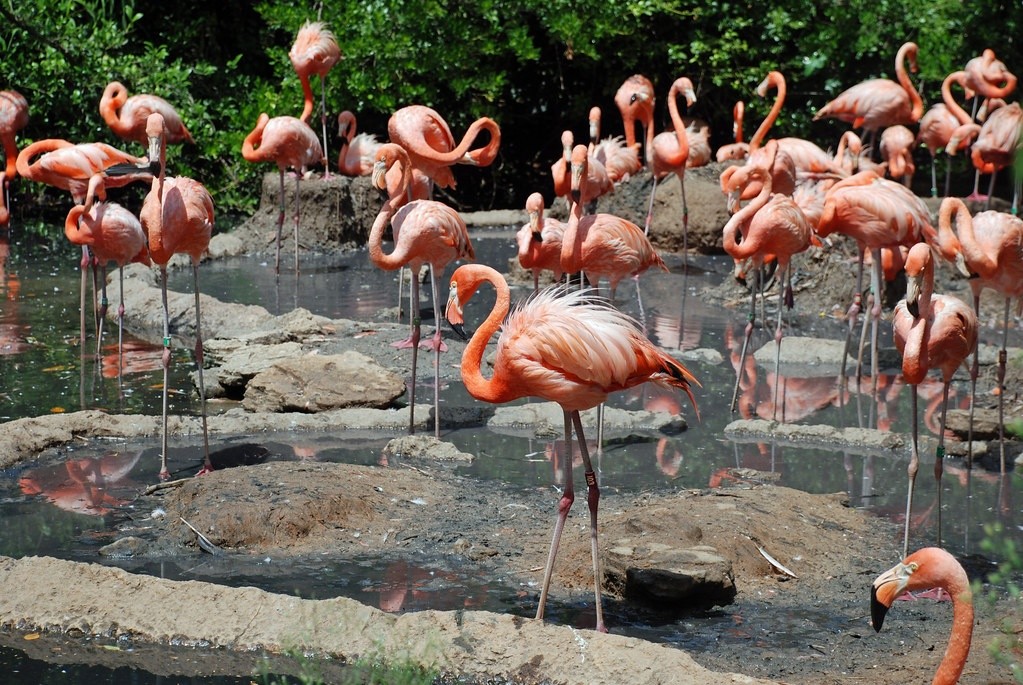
[1,19,1023,684]
